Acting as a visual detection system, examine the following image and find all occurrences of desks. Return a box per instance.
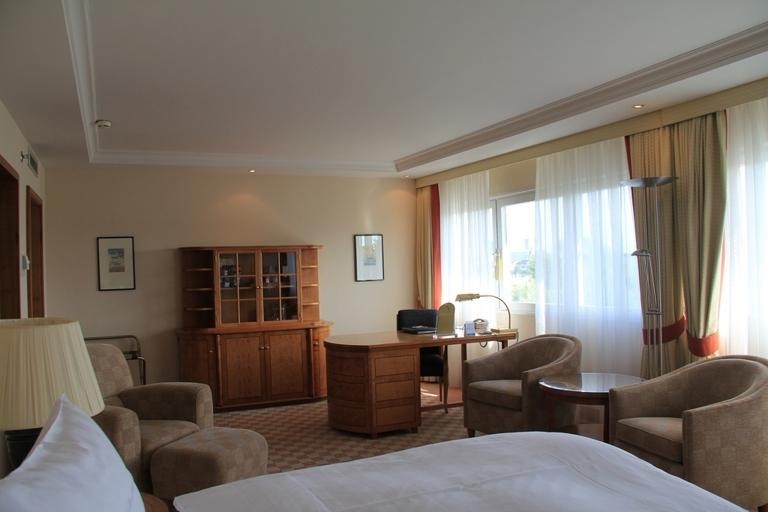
[323,332,516,438]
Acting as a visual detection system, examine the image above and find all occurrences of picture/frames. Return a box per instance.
[354,234,385,282]
[97,236,136,291]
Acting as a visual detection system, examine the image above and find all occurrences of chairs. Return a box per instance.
[462,333,582,437]
[86,343,213,492]
[609,356,768,512]
[396,309,448,418]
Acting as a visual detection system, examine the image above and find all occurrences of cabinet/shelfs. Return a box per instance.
[179,246,323,328]
[174,321,330,411]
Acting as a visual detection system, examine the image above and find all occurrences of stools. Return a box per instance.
[156,425,269,500]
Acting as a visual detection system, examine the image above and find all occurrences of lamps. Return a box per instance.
[0,317,105,433]
[456,294,518,333]
[619,175,678,378]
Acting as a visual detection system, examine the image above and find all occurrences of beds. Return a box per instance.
[0,435,750,512]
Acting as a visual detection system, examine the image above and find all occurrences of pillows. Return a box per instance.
[0,392,145,512]
